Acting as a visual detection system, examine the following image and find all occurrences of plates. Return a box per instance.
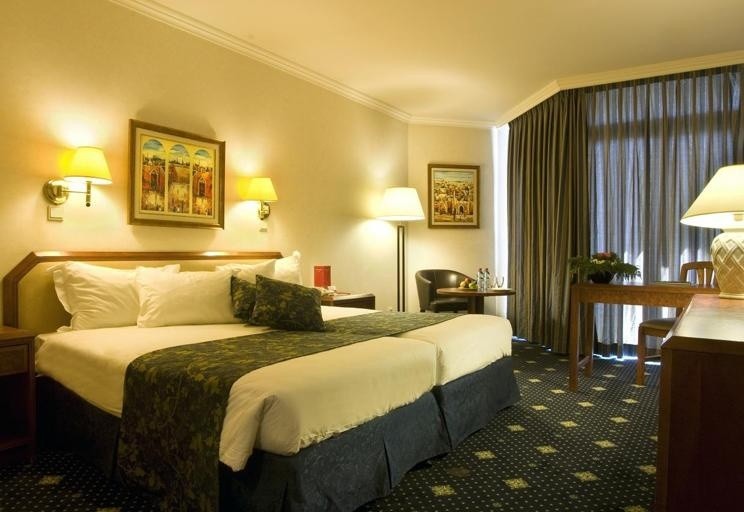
[458,287,476,291]
[491,288,512,292]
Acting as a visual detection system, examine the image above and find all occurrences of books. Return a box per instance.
[655,280,690,285]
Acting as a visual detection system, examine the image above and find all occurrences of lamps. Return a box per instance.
[374,187,425,226]
[43,147,115,210]
[243,176,277,220]
[679,163,744,299]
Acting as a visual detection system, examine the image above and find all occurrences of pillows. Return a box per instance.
[248,272,325,332]
[133,264,245,329]
[272,251,303,284]
[46,260,180,333]
[214,259,279,279]
[228,275,254,319]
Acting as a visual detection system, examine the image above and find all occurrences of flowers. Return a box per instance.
[565,251,641,285]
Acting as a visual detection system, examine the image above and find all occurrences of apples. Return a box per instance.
[460,278,476,289]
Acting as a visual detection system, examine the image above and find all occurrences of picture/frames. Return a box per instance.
[427,162,481,230]
[126,116,226,229]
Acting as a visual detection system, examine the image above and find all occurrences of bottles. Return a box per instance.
[477,268,490,291]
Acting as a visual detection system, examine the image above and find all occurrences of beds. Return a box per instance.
[0,250,520,510]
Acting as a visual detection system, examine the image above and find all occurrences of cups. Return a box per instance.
[490,276,504,287]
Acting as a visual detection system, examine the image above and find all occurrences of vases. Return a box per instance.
[588,272,614,282]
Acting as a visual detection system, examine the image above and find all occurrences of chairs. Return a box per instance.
[415,269,476,312]
[639,260,717,385]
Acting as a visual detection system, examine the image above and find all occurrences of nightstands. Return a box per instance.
[0,322,35,468]
[313,289,376,312]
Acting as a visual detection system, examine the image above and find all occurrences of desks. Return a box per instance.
[568,283,718,394]
[436,286,514,314]
[654,290,744,510]
